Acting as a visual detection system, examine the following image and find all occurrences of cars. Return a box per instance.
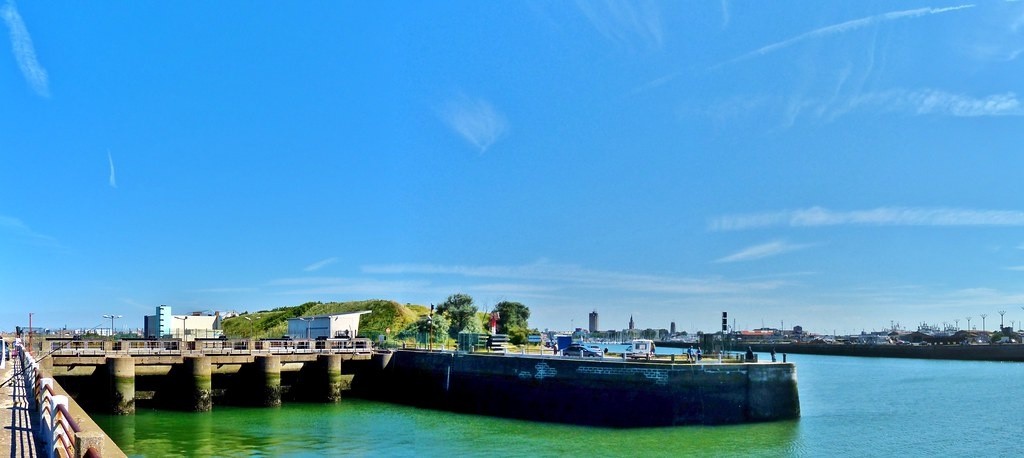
[563,346,597,357]
[622,346,636,359]
[589,345,603,358]
[897,340,928,346]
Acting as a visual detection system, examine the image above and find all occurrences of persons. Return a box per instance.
[687,346,695,362]
[553,342,558,355]
[486,335,492,349]
[649,342,658,357]
[694,347,702,361]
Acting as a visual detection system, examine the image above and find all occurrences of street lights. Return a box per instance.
[942,310,1007,333]
[245,316,261,339]
[103,315,123,341]
